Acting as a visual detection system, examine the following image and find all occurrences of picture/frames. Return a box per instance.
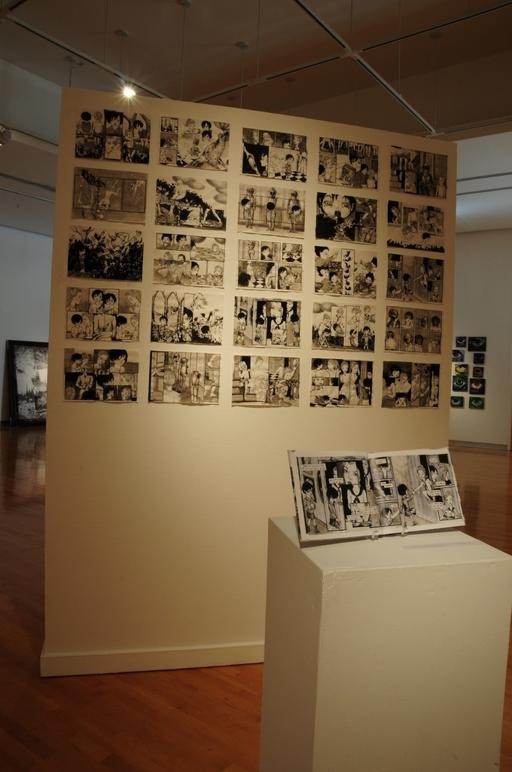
[7,338,49,429]
